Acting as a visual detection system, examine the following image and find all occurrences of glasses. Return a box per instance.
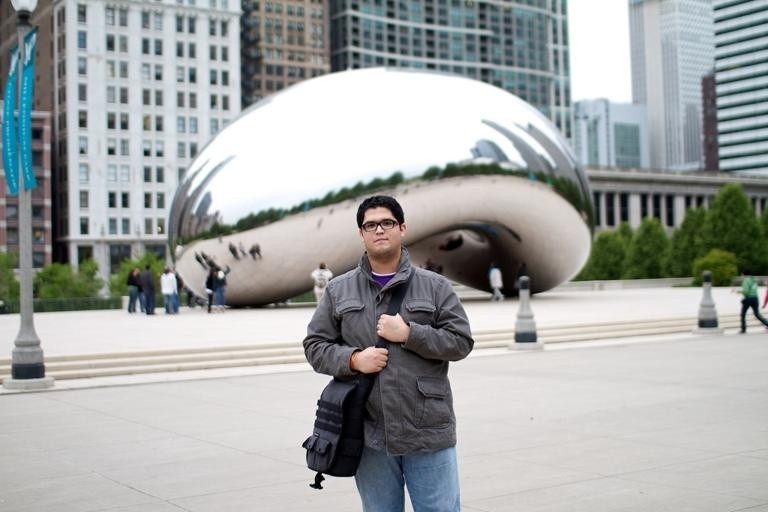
[360,218,400,232]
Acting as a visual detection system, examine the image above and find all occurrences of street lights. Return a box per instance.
[12,0,46,378]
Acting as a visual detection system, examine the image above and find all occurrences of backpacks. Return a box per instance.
[314,270,327,289]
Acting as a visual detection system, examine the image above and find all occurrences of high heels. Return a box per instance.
[217,308,225,314]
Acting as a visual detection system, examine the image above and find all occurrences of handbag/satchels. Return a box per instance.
[301,379,366,489]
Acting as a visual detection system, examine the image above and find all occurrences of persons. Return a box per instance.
[302,193,475,512]
[193,239,263,270]
[731,271,767,333]
[311,263,333,308]
[488,262,504,302]
[125,264,233,315]
